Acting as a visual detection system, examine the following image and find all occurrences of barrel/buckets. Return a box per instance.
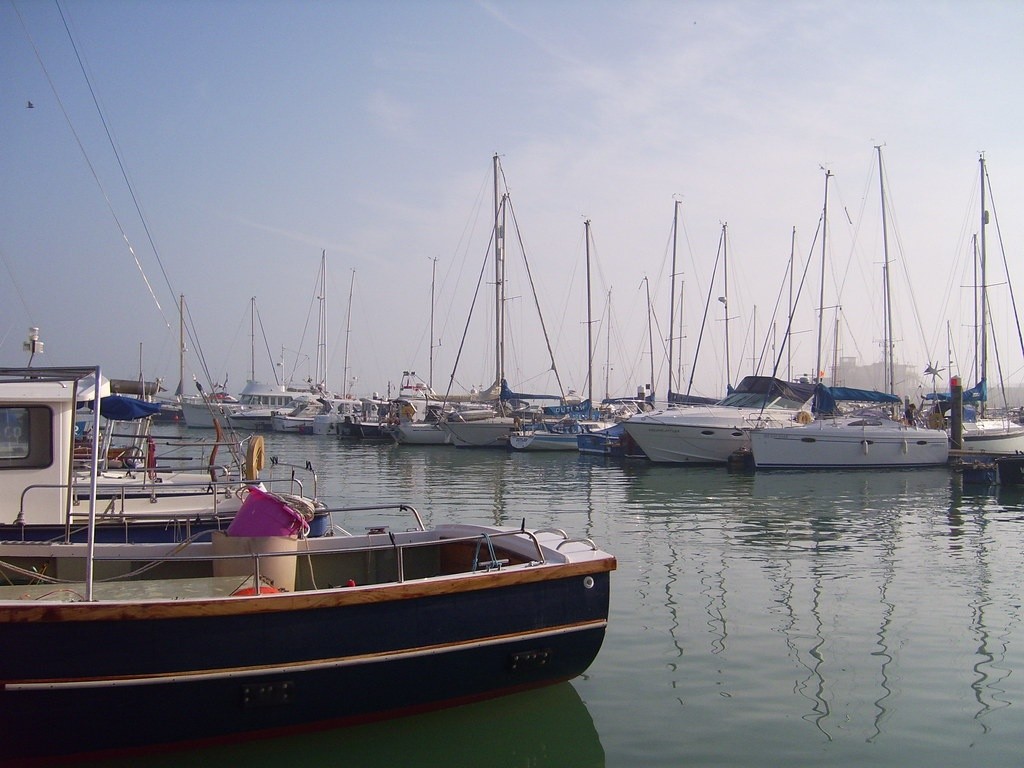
[227,484,310,538]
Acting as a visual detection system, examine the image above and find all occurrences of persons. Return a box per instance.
[904,404,916,424]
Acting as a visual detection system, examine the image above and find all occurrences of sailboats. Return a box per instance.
[1,1,1024,767]
